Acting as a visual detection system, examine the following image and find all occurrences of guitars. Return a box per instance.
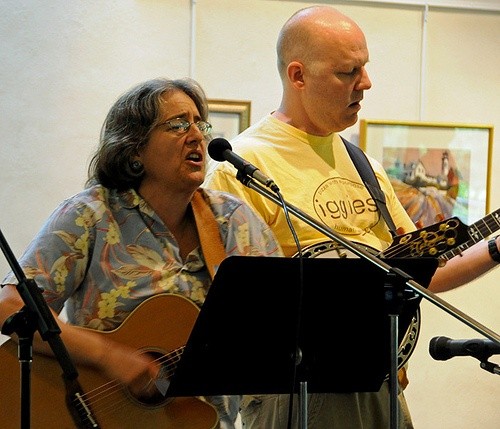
[0,214,471,429]
[290,208,500,383]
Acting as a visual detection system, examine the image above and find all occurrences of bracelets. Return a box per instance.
[487,234,500,265]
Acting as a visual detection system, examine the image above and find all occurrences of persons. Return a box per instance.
[0,77,288,428]
[198,5,500,429]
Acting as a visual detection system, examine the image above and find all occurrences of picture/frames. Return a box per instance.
[207,101,250,163]
[360,119,494,241]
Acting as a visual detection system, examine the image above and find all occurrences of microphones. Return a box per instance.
[208,138,280,193]
[428,336,500,360]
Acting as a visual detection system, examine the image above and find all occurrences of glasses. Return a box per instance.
[155,117,212,137]
